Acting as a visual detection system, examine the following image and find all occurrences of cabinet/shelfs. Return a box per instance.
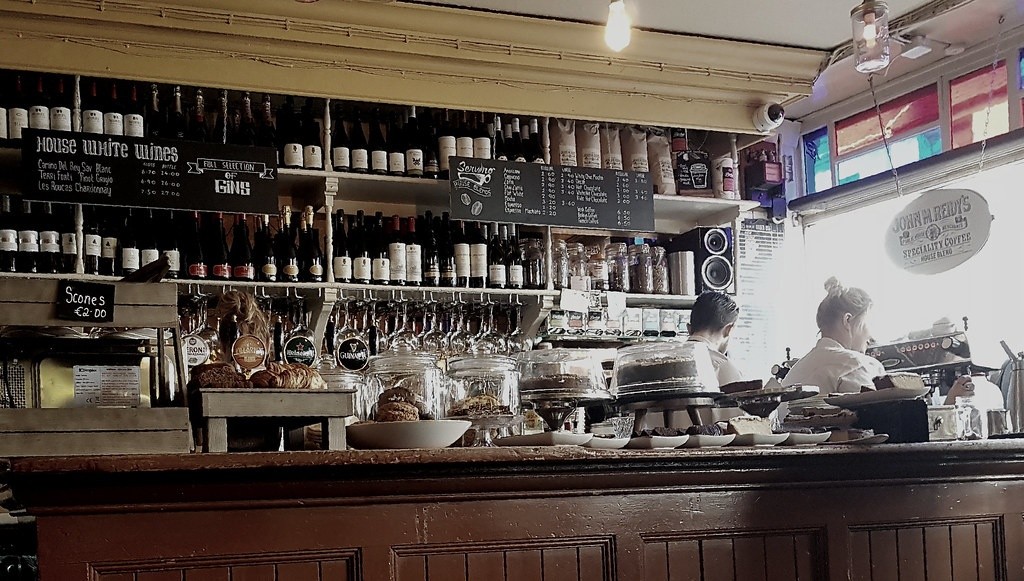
[0,70,761,388]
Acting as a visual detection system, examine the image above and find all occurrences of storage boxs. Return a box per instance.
[856,406,959,442]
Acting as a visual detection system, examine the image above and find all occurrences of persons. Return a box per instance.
[779,276,974,423]
[644,291,744,426]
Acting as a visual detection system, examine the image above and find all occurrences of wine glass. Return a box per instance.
[178,292,532,363]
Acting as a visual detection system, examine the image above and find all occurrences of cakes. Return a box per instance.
[616,356,703,393]
[518,372,597,394]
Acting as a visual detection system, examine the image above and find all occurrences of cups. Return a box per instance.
[986,408,1006,436]
[711,158,734,199]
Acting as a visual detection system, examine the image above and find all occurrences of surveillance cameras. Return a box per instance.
[753,102,784,131]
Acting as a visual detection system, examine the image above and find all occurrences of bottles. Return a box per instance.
[0,70,670,294]
[968,372,1005,439]
[309,344,521,423]
[1005,359,1024,434]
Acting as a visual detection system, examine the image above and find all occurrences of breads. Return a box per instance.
[630,418,773,438]
[372,387,503,422]
[860,372,925,392]
[250,362,329,389]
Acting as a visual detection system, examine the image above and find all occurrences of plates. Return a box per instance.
[493,431,594,446]
[823,385,931,406]
[684,434,737,449]
[584,435,630,449]
[630,434,690,450]
[735,434,789,447]
[785,431,832,446]
[347,420,472,448]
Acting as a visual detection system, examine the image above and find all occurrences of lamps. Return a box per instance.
[850,0,890,74]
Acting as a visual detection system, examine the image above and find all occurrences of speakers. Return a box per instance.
[674,227,735,295]
